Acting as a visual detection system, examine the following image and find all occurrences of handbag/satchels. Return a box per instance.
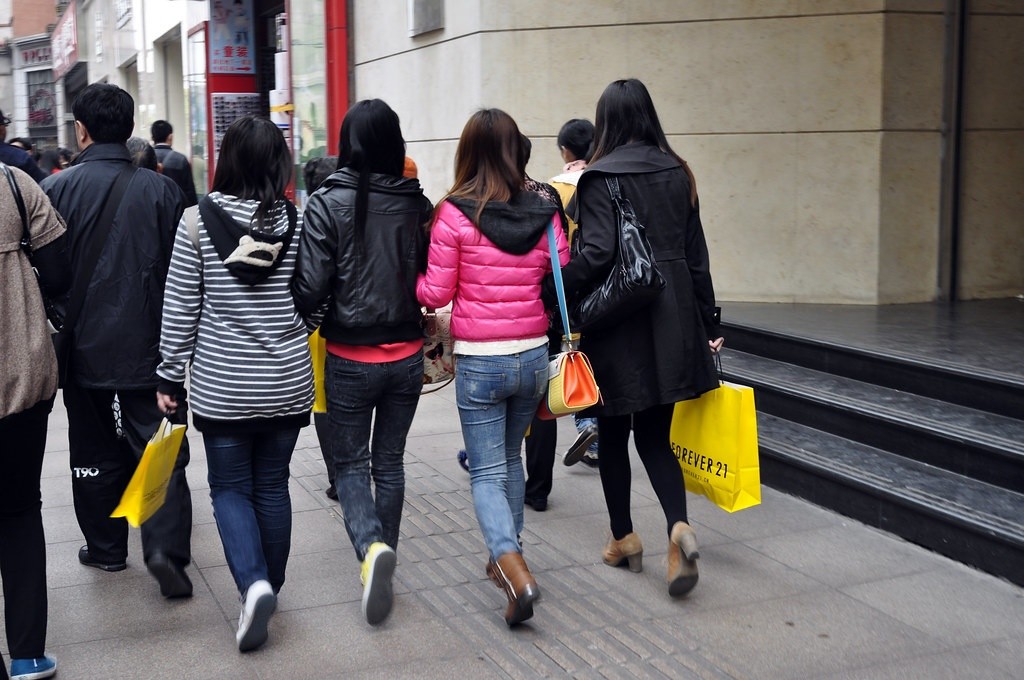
[110,402,188,528]
[565,167,668,329]
[310,325,327,413]
[671,346,763,514]
[422,307,455,395]
[39,292,77,375]
[538,349,605,420]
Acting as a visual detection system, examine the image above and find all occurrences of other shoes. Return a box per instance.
[358,542,397,626]
[79,545,128,572]
[236,579,275,654]
[562,427,599,467]
[522,488,547,513]
[146,547,194,598]
[581,451,599,467]
[456,449,470,473]
[326,486,336,498]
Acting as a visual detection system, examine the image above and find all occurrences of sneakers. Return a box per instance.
[10,652,56,680]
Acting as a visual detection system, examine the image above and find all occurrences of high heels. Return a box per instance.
[601,530,643,572]
[667,521,699,599]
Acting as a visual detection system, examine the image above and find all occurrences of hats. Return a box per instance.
[404,157,417,181]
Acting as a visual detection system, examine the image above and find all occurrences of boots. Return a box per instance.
[490,550,541,626]
[484,559,502,589]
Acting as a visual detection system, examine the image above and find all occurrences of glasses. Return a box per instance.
[0,117,11,129]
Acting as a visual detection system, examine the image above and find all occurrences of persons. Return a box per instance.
[0,109,49,184]
[540,78,725,597]
[151,120,199,204]
[30,83,194,598]
[416,107,571,625]
[0,161,68,680]
[7,137,32,157]
[39,150,66,174]
[457,137,569,512]
[304,156,337,500]
[547,119,599,467]
[157,115,329,653]
[289,98,434,631]
[58,147,80,169]
[127,137,162,172]
